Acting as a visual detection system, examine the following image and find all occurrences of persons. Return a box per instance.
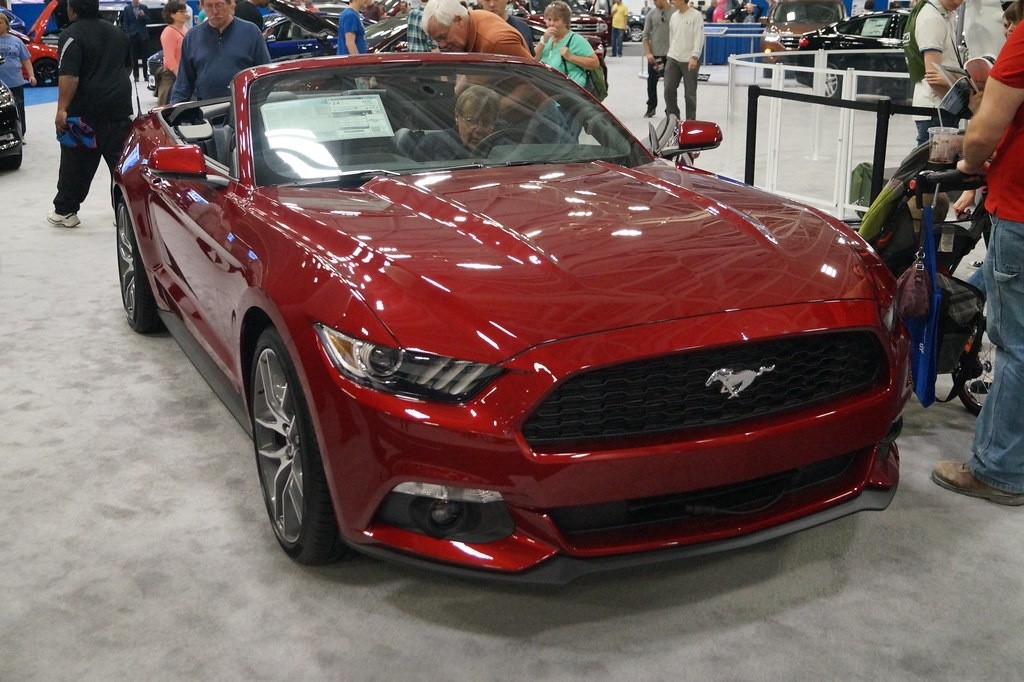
[663,0,705,120]
[194,1,206,25]
[407,0,437,52]
[609,0,628,57]
[644,0,676,117]
[640,1,652,15]
[159,0,189,76]
[47,0,134,227]
[534,0,600,144]
[365,0,408,22]
[904,0,984,145]
[179,0,193,30]
[412,85,516,165]
[0,13,37,144]
[295,0,319,12]
[169,0,271,109]
[931,0,1024,506]
[421,0,556,144]
[122,0,150,82]
[235,0,274,39]
[337,0,372,56]
[477,0,536,56]
[705,0,764,25]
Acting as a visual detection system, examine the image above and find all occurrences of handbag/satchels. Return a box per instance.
[896,211,930,317]
[907,207,942,408]
[562,33,608,103]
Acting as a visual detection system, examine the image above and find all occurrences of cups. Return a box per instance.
[927,126,959,165]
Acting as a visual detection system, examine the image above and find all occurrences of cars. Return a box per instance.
[723,0,764,24]
[0,0,645,88]
[794,8,916,104]
[757,0,850,79]
[0,55,23,169]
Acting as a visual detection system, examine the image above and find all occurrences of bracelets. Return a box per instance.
[540,36,546,45]
[692,56,698,59]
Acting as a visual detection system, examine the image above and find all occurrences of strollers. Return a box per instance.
[857,123,996,417]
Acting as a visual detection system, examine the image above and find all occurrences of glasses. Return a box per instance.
[176,11,186,14]
[548,3,564,10]
[661,10,665,22]
[201,2,228,12]
[461,114,495,129]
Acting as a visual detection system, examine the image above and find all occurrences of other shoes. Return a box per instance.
[619,53,621,57]
[610,54,616,57]
[645,108,655,118]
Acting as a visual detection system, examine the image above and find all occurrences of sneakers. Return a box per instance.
[932,460,1024,507]
[47,210,80,227]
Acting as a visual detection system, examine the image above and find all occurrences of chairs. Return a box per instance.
[394,98,453,162]
[222,91,339,189]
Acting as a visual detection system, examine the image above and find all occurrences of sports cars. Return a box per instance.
[110,55,915,587]
